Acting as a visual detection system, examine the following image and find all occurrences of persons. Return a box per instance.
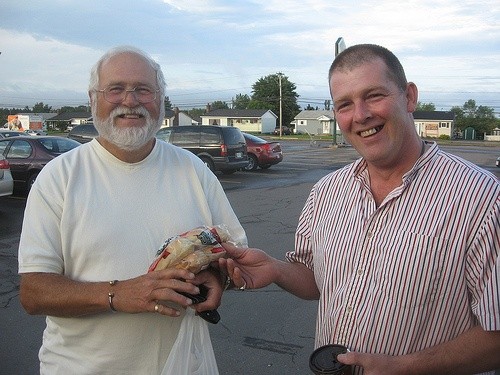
[212,44,500,375]
[19,46,249,375]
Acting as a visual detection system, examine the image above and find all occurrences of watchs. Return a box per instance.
[224,274,231,290]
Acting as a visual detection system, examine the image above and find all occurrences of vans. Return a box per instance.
[156,125,249,174]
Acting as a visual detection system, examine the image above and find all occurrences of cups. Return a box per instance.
[308,345,354,375]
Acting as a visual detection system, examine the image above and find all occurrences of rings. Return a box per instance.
[155,304,159,311]
[240,282,246,291]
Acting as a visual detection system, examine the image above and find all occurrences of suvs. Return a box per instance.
[273,126,290,135]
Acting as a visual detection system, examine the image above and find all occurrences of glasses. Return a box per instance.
[90,85,160,105]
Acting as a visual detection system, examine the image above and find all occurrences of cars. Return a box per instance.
[67,123,99,143]
[25,129,46,136]
[240,130,283,172]
[0,131,82,197]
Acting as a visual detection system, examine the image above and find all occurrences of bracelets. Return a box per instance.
[108,280,117,312]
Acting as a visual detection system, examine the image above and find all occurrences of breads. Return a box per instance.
[150,226,236,275]
